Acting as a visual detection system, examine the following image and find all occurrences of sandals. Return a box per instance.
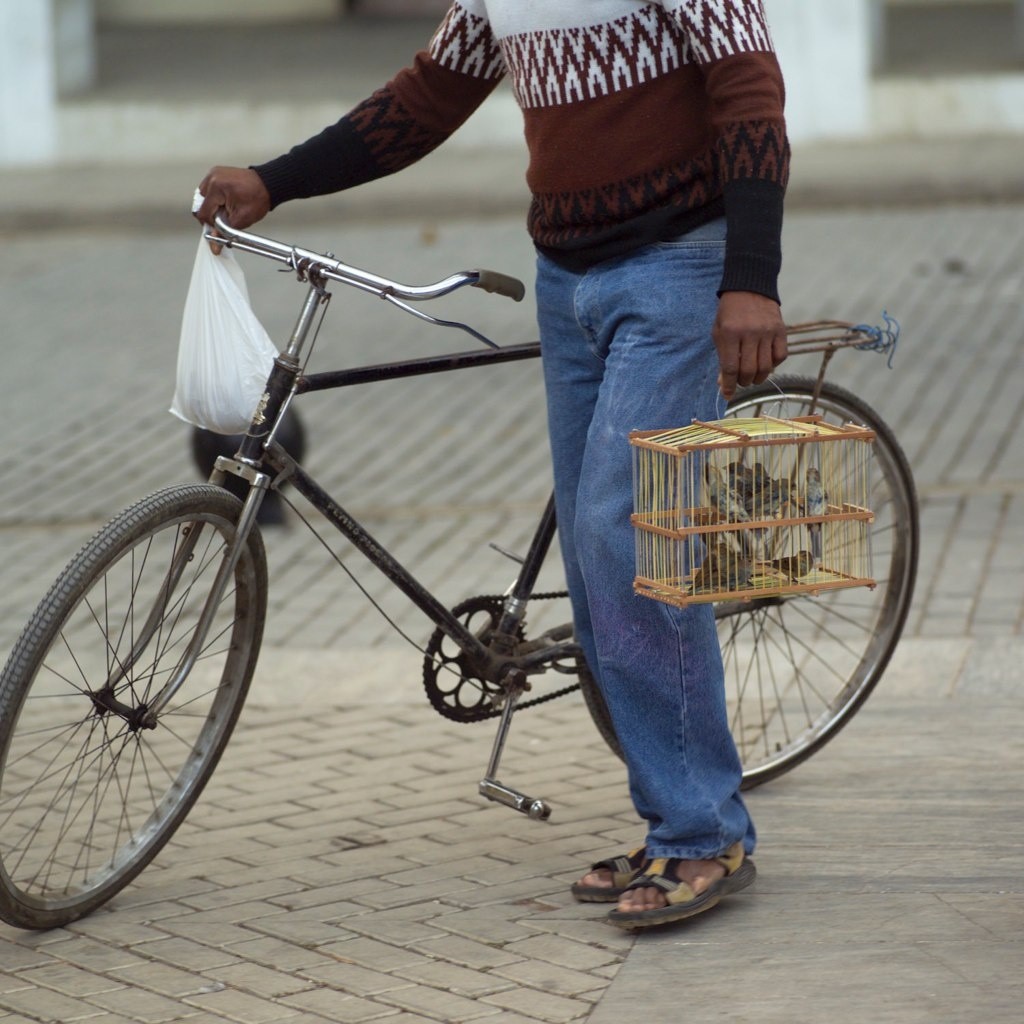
[605,843,756,926]
[569,843,651,901]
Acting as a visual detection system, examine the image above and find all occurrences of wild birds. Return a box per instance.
[686,463,830,593]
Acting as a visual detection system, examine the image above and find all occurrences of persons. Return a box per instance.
[192,0,794,929]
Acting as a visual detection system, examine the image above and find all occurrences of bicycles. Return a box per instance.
[0,189,921,930]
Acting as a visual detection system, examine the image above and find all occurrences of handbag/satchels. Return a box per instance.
[170,190,280,436]
